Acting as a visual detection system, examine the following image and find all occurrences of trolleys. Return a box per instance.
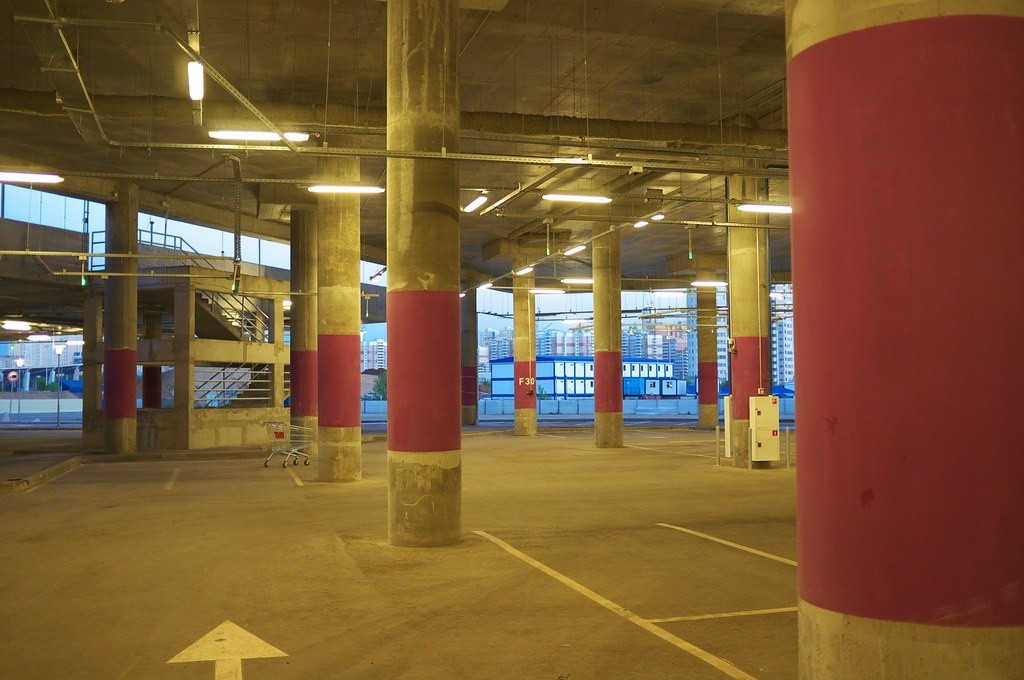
[261,421,315,468]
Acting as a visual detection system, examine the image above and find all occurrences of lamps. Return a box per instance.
[208,85,311,142]
[737,151,792,214]
[307,142,386,194]
[541,185,614,204]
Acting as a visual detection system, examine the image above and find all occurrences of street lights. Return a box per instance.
[15,358,24,423]
[53,344,63,427]
